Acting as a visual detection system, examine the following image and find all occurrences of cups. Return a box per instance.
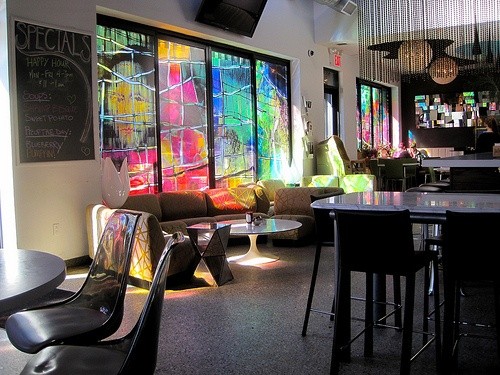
[245,212,254,224]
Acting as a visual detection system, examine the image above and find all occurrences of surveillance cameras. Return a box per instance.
[308,50,314,57]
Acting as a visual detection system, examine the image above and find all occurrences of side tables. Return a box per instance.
[186,223,234,288]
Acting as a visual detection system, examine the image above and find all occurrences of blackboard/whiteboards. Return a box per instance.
[10,15,100,167]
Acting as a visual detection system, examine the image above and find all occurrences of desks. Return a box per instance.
[310,191,500,329]
[422,152,500,194]
[0,248,67,314]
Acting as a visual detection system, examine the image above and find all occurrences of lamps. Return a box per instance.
[335,0,358,17]
[328,48,343,69]
[99,157,131,210]
[356,0,500,84]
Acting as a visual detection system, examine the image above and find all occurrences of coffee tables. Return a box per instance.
[215,218,303,266]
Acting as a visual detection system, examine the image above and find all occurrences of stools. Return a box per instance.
[300,171,500,375]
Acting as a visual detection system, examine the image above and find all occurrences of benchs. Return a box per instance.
[369,157,421,187]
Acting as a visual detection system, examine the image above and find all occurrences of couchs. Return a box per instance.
[86,180,343,291]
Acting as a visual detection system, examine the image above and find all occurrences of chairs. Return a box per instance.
[0,210,185,375]
[376,158,410,192]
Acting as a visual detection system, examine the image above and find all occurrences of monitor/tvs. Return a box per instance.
[194,0,268,37]
